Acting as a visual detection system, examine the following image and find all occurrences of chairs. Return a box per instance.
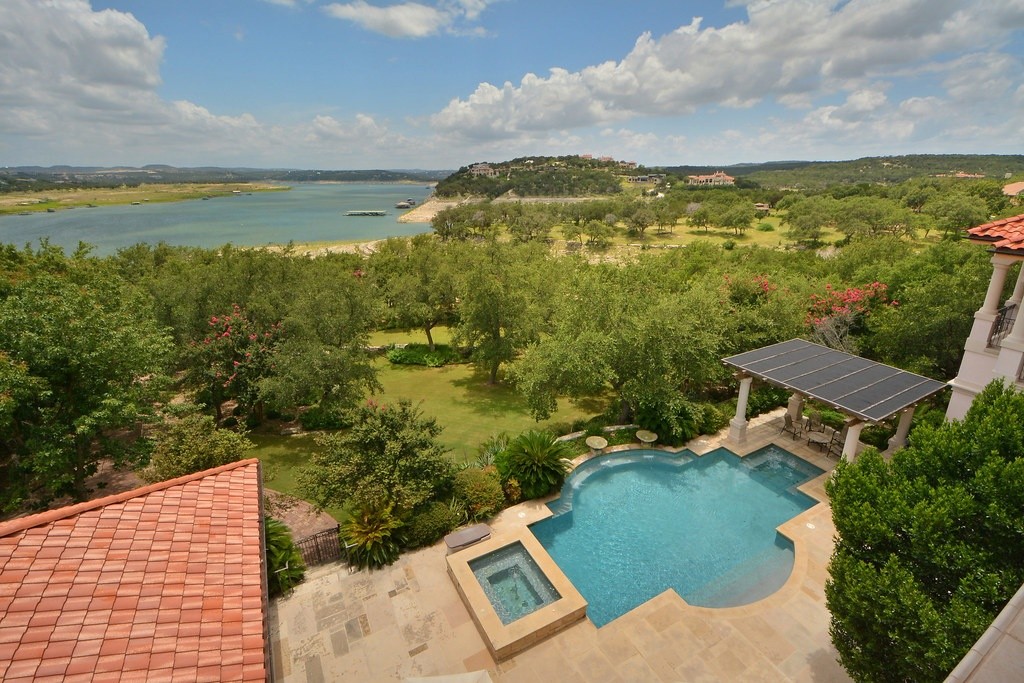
[780,413,802,441]
[805,412,825,433]
[827,430,847,457]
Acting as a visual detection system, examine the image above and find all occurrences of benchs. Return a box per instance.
[443,523,490,549]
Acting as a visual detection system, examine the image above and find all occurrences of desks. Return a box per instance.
[807,431,831,452]
